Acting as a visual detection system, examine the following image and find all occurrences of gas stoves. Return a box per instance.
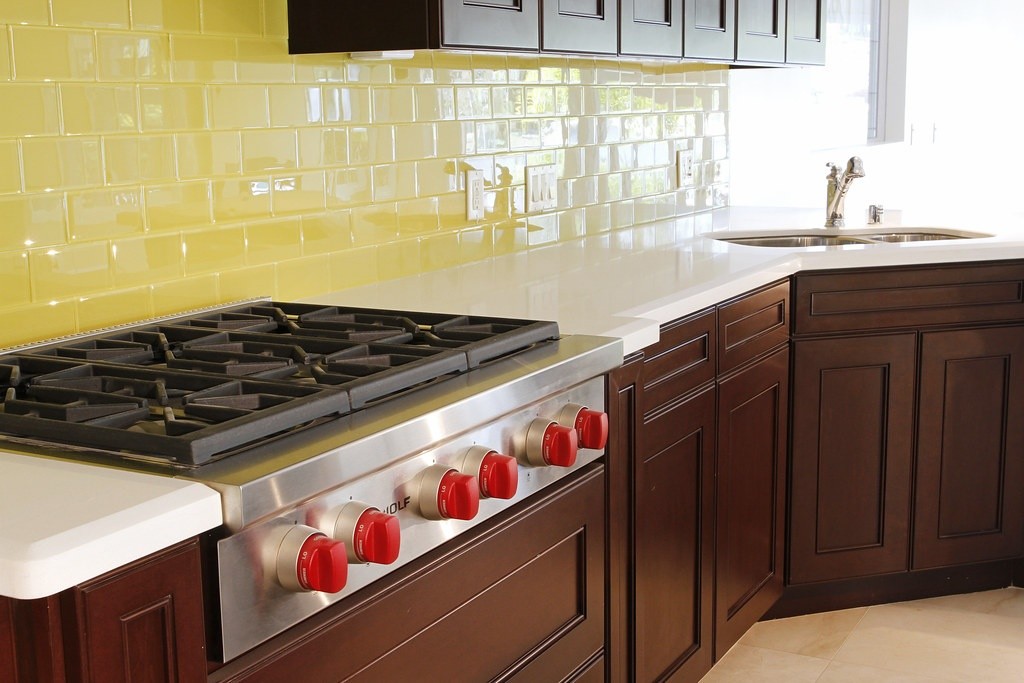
[0,299,625,667]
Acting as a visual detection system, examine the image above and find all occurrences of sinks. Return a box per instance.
[715,235,877,248]
[868,233,972,244]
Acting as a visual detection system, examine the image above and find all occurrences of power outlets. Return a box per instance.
[677,149,694,188]
[466,170,485,220]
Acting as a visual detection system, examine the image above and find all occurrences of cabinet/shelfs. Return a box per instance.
[207,463,606,683]
[629,276,785,683]
[785,256,1023,587]
[288,0,827,68]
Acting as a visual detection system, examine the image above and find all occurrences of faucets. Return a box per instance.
[824,156,866,228]
